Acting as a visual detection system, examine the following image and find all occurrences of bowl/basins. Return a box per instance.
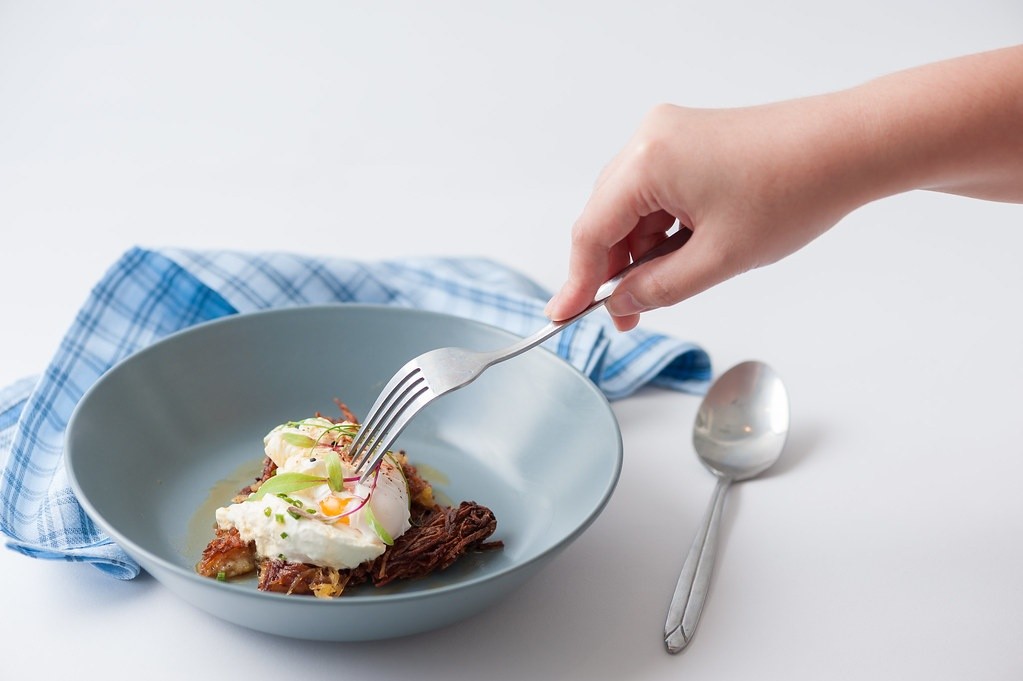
[64,302,625,642]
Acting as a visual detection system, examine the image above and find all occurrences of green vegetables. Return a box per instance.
[246,419,409,546]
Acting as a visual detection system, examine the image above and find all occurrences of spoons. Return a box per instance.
[661,360,791,656]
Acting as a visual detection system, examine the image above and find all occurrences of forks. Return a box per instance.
[347,226,692,485]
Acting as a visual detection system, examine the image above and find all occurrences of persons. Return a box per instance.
[545,46,1019,332]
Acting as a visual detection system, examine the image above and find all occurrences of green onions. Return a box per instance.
[217,492,317,582]
